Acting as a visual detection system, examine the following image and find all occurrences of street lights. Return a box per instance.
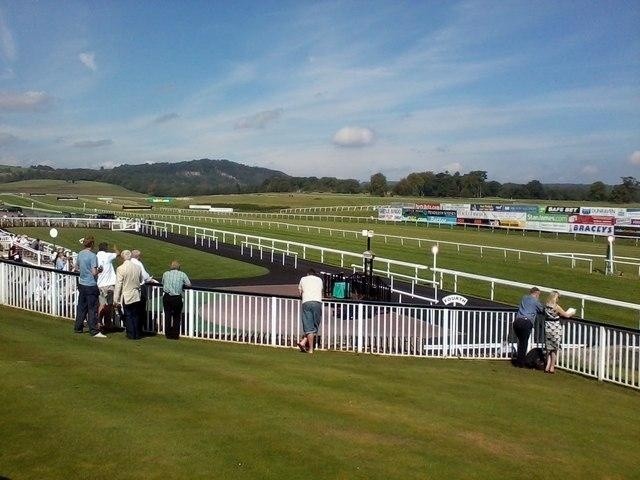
[431,246,439,304]
[361,230,374,285]
[49,228,58,250]
[607,235,615,274]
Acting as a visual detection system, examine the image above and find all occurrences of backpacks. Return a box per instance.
[526,348,546,370]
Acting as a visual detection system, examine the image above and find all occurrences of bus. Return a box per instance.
[97,213,114,219]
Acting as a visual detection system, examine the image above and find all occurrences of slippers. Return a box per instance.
[297,343,307,352]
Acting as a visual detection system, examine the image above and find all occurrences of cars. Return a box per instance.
[3,206,22,212]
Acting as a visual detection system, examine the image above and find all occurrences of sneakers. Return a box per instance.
[94,332,107,338]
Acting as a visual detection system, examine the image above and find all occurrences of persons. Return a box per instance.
[0,231,44,262]
[544,292,577,374]
[161,261,192,340]
[297,268,323,355]
[513,287,546,367]
[49,234,160,340]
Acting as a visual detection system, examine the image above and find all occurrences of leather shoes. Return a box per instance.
[513,358,524,367]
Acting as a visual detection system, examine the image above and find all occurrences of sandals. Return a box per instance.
[544,367,555,373]
